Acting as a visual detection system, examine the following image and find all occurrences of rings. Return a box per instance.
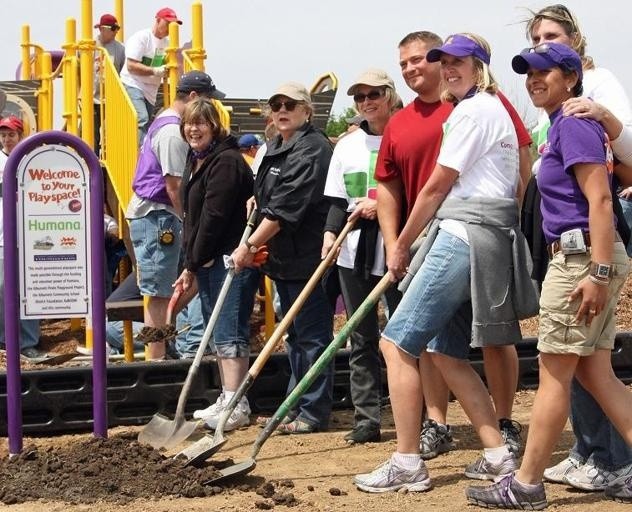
[588,308,597,313]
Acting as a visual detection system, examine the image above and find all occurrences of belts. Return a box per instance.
[542,225,624,254]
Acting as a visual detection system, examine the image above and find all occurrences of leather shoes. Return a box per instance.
[274,418,317,435]
[255,412,292,429]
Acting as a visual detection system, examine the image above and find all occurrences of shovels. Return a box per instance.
[136,283,184,342]
[173,207,362,470]
[137,197,258,453]
[204,234,429,485]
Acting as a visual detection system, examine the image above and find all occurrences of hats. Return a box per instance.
[425,34,490,65]
[176,69,226,101]
[510,43,585,78]
[0,115,24,134]
[267,81,312,107]
[94,13,117,28]
[154,7,183,25]
[347,68,395,98]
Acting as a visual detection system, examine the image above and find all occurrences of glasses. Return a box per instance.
[183,118,211,127]
[100,25,120,32]
[353,90,386,104]
[268,100,298,113]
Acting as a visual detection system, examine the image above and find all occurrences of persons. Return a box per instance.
[320,68,426,442]
[328,113,364,143]
[374,31,533,460]
[465,42,632,511]
[125,70,226,362]
[239,135,265,168]
[171,95,260,432]
[520,5,632,500]
[0,115,50,363]
[103,215,119,295]
[76,273,216,358]
[352,32,541,494]
[78,15,125,159]
[119,7,183,149]
[229,81,341,434]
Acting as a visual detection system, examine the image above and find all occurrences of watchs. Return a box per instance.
[244,239,258,255]
[590,261,617,278]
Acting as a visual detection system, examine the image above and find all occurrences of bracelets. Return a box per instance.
[586,273,610,286]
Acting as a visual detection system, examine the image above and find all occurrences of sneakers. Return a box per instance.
[498,416,529,468]
[564,464,631,491]
[465,473,549,511]
[353,453,433,493]
[543,456,586,485]
[464,452,520,482]
[602,474,631,504]
[191,393,232,420]
[205,406,252,429]
[342,425,382,444]
[417,419,459,460]
